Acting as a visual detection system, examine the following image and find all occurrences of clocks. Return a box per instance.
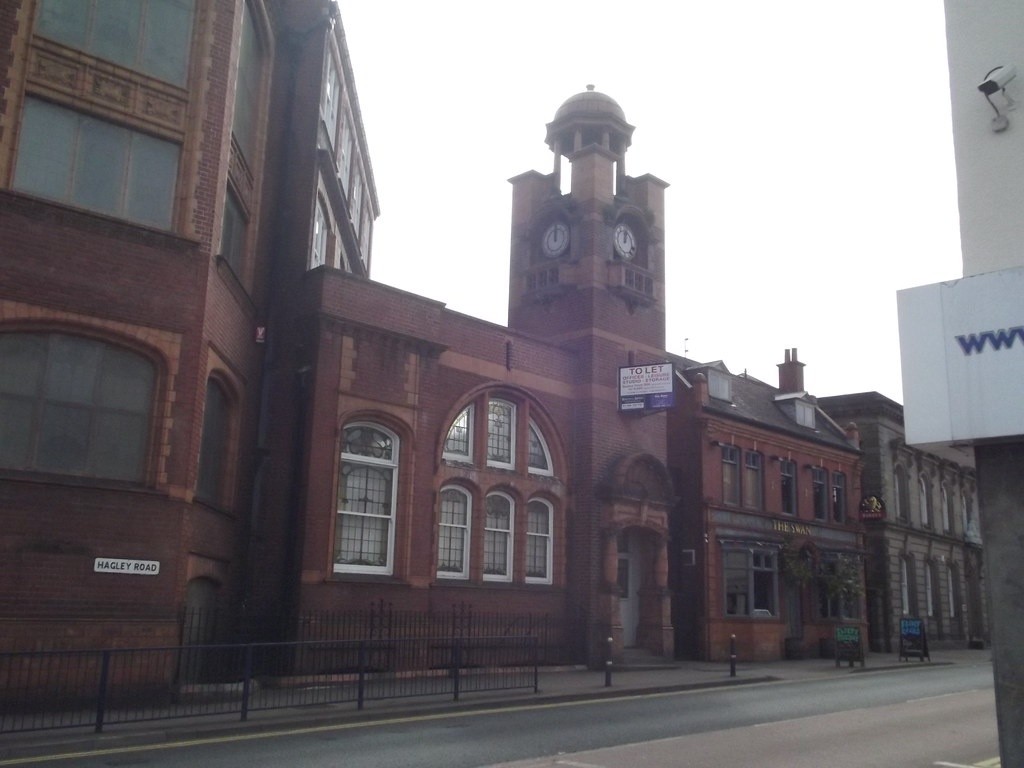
[613,221,637,260]
[542,221,570,257]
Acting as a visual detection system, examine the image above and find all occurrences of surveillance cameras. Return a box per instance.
[977,63,1017,96]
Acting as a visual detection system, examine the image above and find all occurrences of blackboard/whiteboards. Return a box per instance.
[899,618,926,656]
[834,624,865,662]
